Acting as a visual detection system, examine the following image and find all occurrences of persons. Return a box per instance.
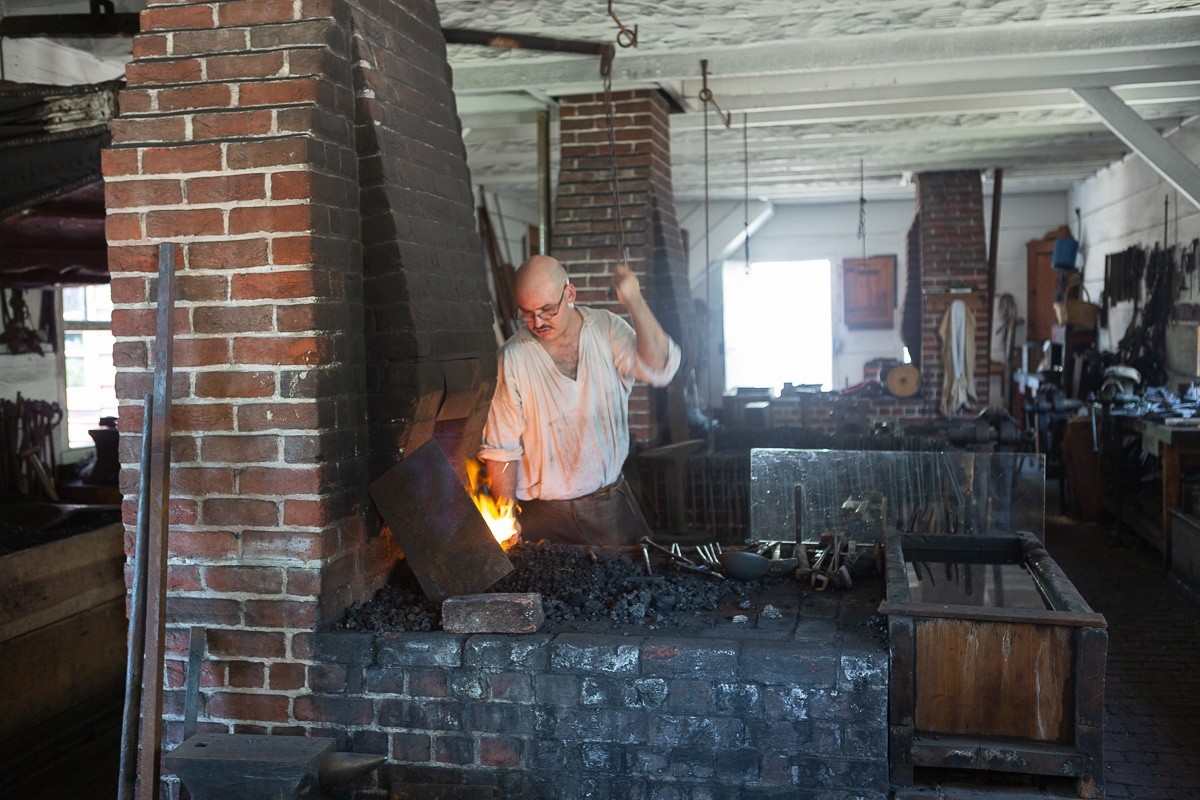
[482,254,681,551]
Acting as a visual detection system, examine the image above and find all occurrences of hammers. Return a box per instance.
[794,544,813,580]
[766,542,798,575]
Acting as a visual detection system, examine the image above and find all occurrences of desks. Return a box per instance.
[1099,413,1200,578]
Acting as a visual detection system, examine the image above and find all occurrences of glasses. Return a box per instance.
[514,284,567,323]
